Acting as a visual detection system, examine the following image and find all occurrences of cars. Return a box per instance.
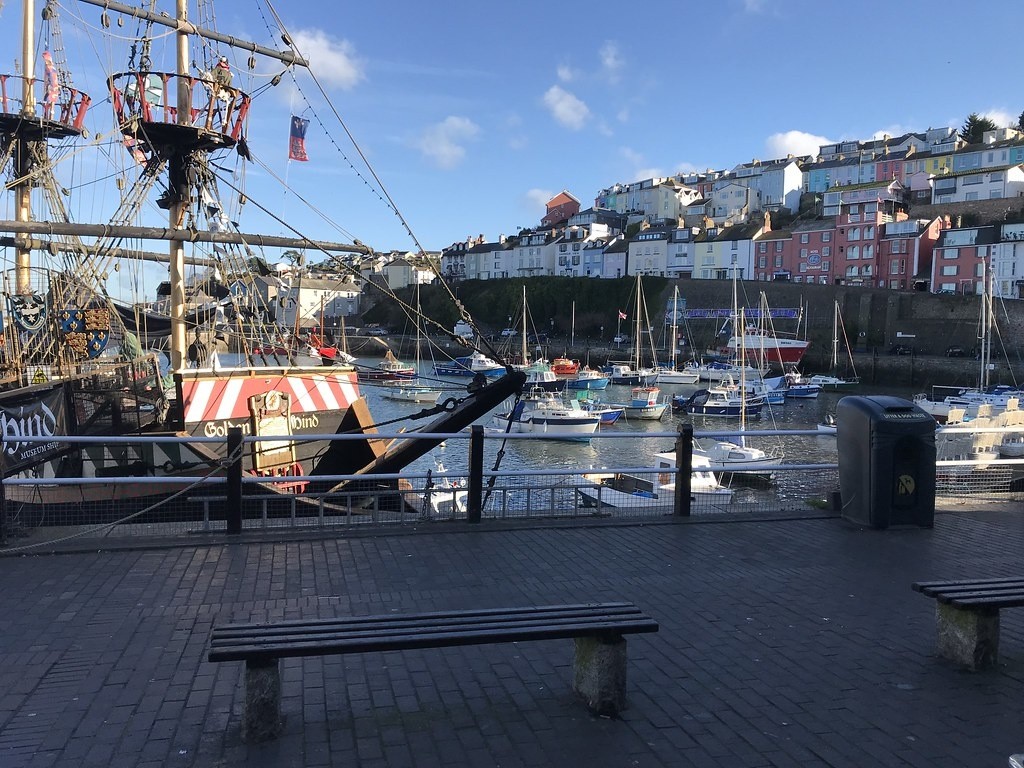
[460,331,475,340]
[482,334,500,343]
[945,345,964,358]
[893,343,911,355]
[526,334,546,344]
[502,328,517,337]
[613,334,632,344]
[367,328,386,336]
[971,342,997,358]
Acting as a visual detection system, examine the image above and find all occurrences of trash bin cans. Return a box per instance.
[836,395,937,529]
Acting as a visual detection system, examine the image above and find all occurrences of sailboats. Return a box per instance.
[0,0,858,512]
[692,305,785,477]
[912,254,1024,494]
[379,276,444,405]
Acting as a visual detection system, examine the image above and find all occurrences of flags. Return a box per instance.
[616,312,627,320]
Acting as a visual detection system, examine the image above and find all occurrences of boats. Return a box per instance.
[577,447,735,508]
[592,408,625,425]
[491,388,600,442]
[577,382,670,422]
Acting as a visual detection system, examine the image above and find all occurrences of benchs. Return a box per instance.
[208,601,660,745]
[911,577,1024,668]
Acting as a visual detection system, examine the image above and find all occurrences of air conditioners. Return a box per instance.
[944,238,953,245]
